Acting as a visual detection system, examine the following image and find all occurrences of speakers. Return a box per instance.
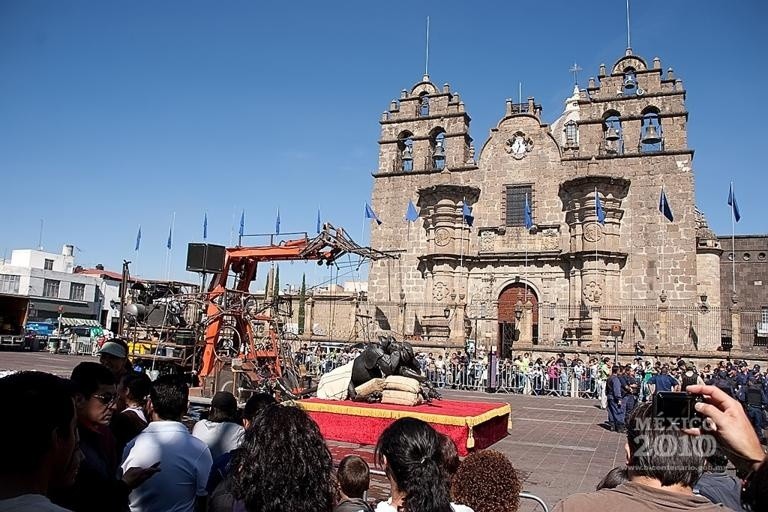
[186,243,225,274]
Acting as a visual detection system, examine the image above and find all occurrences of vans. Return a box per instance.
[55,326,105,347]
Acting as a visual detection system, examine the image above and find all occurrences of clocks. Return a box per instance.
[504,130,534,160]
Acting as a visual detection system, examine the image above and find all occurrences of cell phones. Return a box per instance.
[149,461,161,469]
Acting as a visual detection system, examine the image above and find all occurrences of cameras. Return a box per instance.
[651,390,704,428]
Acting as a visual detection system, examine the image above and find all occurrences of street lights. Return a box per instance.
[97,295,103,325]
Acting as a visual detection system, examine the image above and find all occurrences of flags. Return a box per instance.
[403,200,418,223]
[201,211,208,239]
[657,188,673,223]
[238,210,245,237]
[363,203,383,226]
[166,225,172,251]
[316,208,321,235]
[521,198,532,228]
[463,202,474,229]
[133,225,142,251]
[594,190,605,227]
[275,209,280,235]
[725,182,741,224]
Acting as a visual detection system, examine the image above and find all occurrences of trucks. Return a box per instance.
[1,292,35,349]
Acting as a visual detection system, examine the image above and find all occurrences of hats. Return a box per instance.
[738,360,747,367]
[94,341,129,361]
[677,360,684,368]
[686,363,693,370]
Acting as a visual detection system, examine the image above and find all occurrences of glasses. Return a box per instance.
[83,389,122,404]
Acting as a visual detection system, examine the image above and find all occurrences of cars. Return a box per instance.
[26,320,57,336]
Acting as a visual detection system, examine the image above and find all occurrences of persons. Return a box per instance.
[0,339,768,511]
